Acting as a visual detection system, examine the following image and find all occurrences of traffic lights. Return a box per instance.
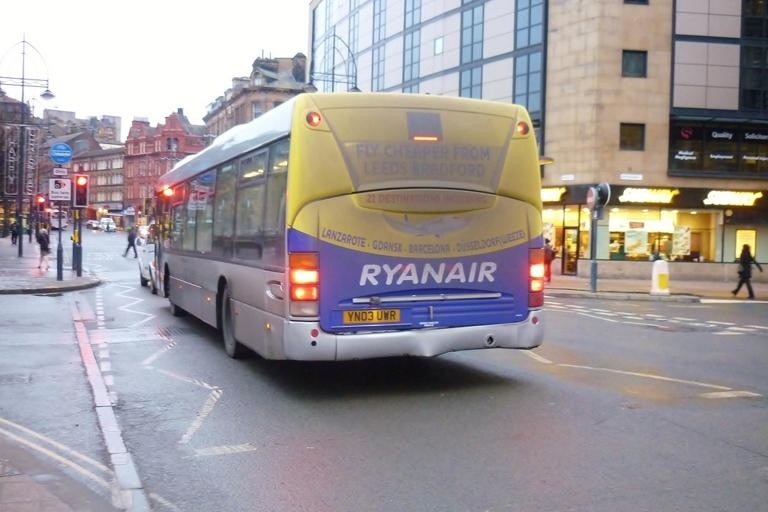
[33,192,48,207]
[76,176,88,205]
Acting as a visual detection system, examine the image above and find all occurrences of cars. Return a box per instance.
[86,218,117,232]
[134,224,155,294]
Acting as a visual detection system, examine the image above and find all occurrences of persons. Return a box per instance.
[35,227,51,270]
[8,220,21,245]
[731,243,764,299]
[543,237,557,284]
[120,226,137,259]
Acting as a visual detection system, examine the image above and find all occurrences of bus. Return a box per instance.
[155,92,546,362]
[47,210,68,229]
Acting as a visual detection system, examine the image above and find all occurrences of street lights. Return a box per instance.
[0,76,55,100]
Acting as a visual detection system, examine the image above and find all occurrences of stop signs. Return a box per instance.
[586,187,596,211]
